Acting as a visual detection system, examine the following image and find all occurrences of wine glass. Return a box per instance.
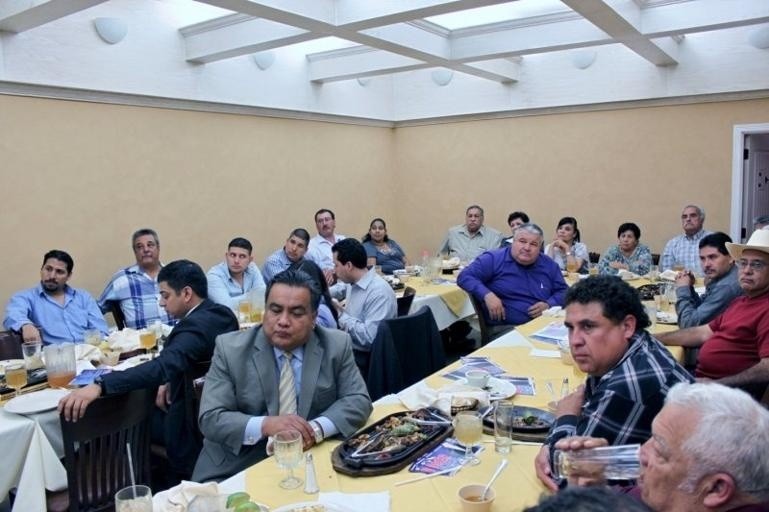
[453,409,484,467]
[272,430,304,491]
[4,361,28,397]
[138,319,162,362]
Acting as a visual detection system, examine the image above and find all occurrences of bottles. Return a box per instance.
[303,453,320,496]
[560,377,570,399]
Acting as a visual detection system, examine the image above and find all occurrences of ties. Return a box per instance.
[279,352,297,415]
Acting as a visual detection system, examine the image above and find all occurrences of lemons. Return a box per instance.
[226,492,250,509]
[233,502,259,512]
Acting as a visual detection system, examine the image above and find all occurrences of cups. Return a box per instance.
[549,441,642,481]
[83,329,101,344]
[383,249,460,289]
[457,483,497,512]
[464,367,490,389]
[493,401,514,455]
[566,258,598,286]
[113,484,154,511]
[233,298,262,324]
[653,284,678,324]
[647,260,684,282]
[21,341,77,388]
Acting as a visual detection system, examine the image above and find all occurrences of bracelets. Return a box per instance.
[565,252,572,256]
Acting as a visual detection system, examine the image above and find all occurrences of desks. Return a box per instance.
[1,260,477,510]
[216,267,708,512]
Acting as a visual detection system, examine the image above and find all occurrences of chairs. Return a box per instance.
[146,354,202,486]
[185,359,212,479]
[346,305,448,403]
[102,298,126,331]
[463,285,520,348]
[392,287,416,317]
[7,387,144,512]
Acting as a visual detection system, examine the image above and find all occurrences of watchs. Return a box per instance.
[307,420,323,444]
[94,376,106,397]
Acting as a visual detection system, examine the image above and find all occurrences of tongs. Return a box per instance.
[347,427,406,460]
[398,405,453,428]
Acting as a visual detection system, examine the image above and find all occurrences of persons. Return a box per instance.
[597,223,654,276]
[205,238,267,311]
[261,228,334,286]
[502,210,544,254]
[535,275,697,494]
[438,207,503,263]
[457,224,573,345]
[94,230,182,330]
[523,486,654,512]
[544,216,590,275]
[661,206,769,385]
[553,382,769,512]
[3,250,109,351]
[359,218,411,275]
[306,208,348,281]
[191,271,374,486]
[315,238,398,385]
[58,261,240,482]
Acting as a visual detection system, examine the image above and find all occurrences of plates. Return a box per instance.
[436,377,518,401]
[477,403,556,433]
[4,388,68,415]
[337,406,450,470]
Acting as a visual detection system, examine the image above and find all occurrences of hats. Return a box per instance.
[725,228,769,257]
